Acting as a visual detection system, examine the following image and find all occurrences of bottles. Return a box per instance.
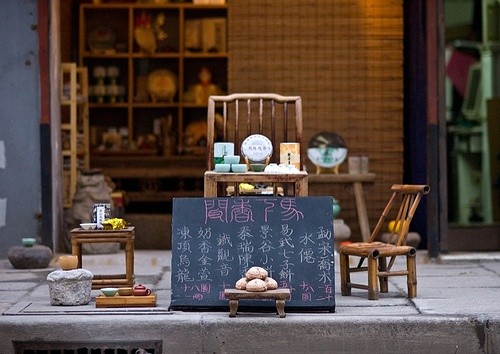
[348,151,361,176]
[333,197,341,218]
[359,151,369,175]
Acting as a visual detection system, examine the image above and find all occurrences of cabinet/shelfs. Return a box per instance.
[61,3,229,207]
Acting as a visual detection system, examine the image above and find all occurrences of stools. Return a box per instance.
[225,289,291,318]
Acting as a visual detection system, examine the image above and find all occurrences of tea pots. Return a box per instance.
[132,284,151,296]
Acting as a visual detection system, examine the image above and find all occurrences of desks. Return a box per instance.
[309,174,375,242]
[70,227,135,289]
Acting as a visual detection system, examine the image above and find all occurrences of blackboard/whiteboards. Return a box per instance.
[170,196,335,311]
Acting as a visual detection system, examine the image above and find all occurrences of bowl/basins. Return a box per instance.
[119,288,132,296]
[264,163,300,174]
[215,164,230,173]
[224,155,240,165]
[101,288,117,296]
[80,223,97,230]
[59,255,78,270]
[231,164,247,173]
[22,238,36,246]
[250,163,265,172]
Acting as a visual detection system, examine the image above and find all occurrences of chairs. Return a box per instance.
[204,92,308,197]
[340,184,430,300]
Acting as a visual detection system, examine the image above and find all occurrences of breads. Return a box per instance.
[235,266,278,291]
